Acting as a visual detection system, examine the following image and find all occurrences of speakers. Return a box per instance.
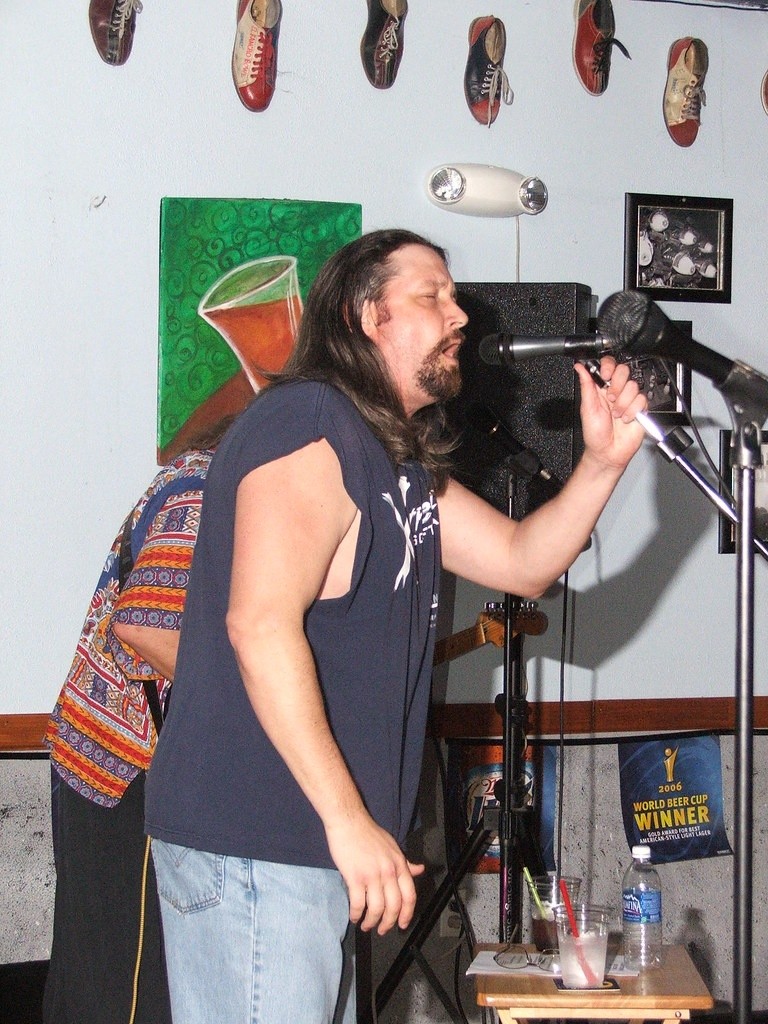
[446,283,594,554]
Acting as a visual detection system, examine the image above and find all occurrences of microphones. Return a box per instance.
[464,402,563,494]
[478,332,611,366]
[598,291,768,410]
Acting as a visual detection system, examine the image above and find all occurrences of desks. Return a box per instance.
[472,942,713,1024]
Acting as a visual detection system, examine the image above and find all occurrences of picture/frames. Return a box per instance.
[589,316,693,426]
[624,193,733,304]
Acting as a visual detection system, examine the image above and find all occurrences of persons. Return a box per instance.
[41,413,238,1024]
[145,228,648,1023]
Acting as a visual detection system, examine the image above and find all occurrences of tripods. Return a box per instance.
[371,593,550,1024]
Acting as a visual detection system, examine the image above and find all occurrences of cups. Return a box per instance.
[525,876,583,953]
[551,904,613,988]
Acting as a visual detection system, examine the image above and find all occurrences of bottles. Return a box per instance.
[622,845,663,969]
